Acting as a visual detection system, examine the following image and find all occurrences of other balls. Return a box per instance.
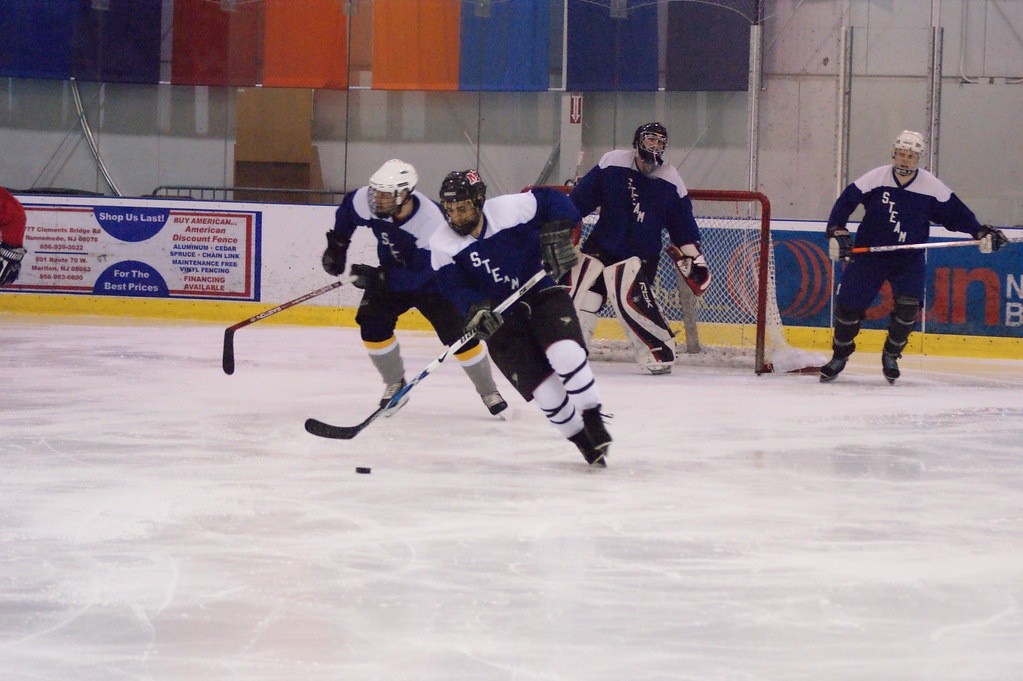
[356,466,371,474]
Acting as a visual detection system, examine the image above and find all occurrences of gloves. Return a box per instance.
[351,264,377,289]
[463,309,504,339]
[322,230,350,276]
[0,242,27,286]
[973,225,1008,254]
[829,227,855,265]
[539,220,578,280]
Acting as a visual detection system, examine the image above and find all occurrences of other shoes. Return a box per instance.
[646,352,677,374]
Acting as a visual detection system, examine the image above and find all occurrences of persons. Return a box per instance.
[569,123,712,375]
[429,169,613,470]
[322,159,508,422]
[0,186,27,288]
[820,131,1008,384]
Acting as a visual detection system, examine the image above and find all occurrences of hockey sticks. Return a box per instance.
[850,236,1023,254]
[304,265,552,441]
[222,275,356,376]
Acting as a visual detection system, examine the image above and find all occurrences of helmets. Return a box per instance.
[633,121,668,161]
[892,129,925,175]
[367,159,417,217]
[439,170,486,236]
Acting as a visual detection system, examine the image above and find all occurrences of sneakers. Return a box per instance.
[481,388,508,421]
[819,356,847,382]
[569,429,608,468]
[381,378,409,417]
[583,405,613,456]
[882,350,901,384]
